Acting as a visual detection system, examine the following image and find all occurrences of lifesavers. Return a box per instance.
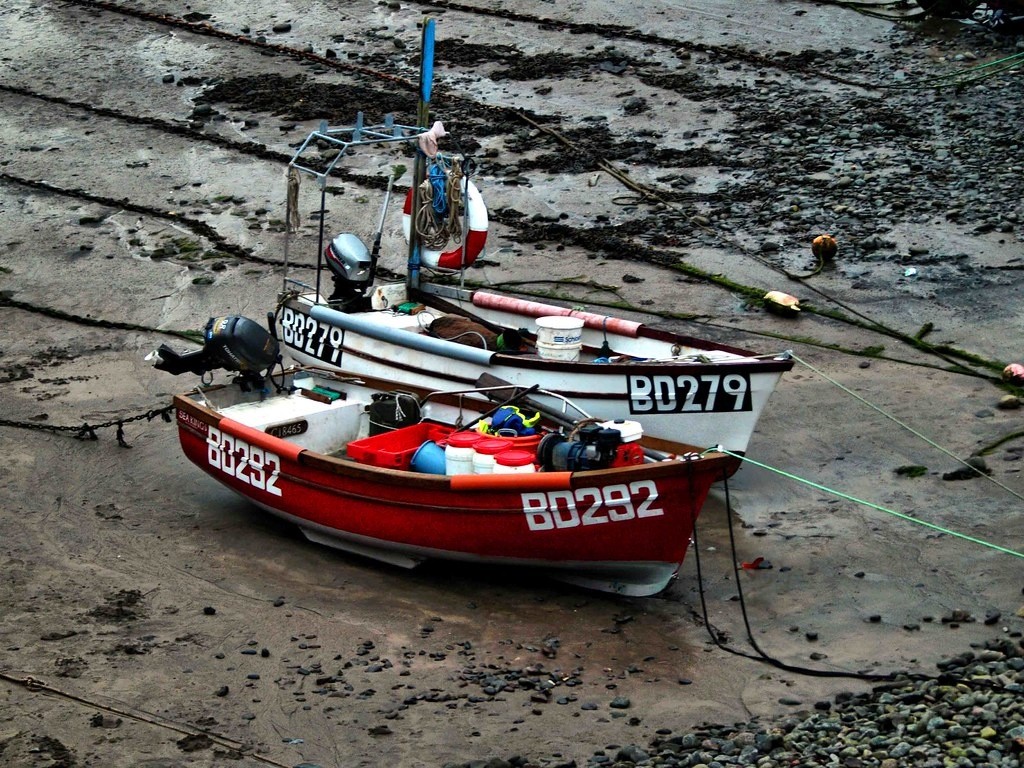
[399,166,489,270]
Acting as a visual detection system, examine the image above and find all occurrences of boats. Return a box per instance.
[144,315,736,601]
[267,15,795,483]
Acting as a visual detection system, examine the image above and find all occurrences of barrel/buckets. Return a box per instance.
[476,422,549,465]
[445,432,483,475]
[471,438,514,475]
[535,316,586,362]
[410,440,446,474]
[497,328,522,350]
[493,450,537,473]
